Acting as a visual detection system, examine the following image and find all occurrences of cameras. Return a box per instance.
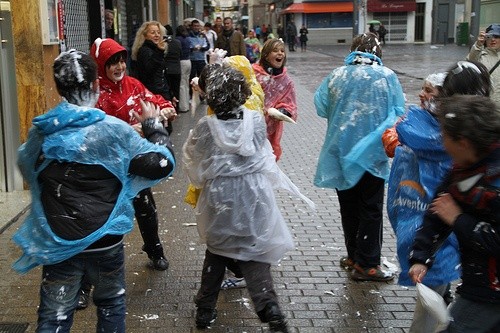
[484,33,493,40]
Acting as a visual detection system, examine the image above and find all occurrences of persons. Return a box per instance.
[105,9,116,39]
[466,24,500,105]
[382,61,500,333]
[182,37,315,333]
[369,24,377,35]
[377,24,388,45]
[14,49,177,333]
[78,38,177,309]
[261,24,272,40]
[313,33,405,281]
[130,17,262,115]
[277,18,308,52]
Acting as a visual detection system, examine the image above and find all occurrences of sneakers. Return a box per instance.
[219,275,247,290]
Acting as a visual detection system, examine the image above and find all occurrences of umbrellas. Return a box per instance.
[368,20,380,25]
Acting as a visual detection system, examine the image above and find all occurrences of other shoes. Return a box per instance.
[148,255,168,271]
[269,316,300,333]
[196,309,216,328]
[76,285,91,310]
[340,257,394,281]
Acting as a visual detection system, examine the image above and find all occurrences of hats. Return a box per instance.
[485,24,500,37]
